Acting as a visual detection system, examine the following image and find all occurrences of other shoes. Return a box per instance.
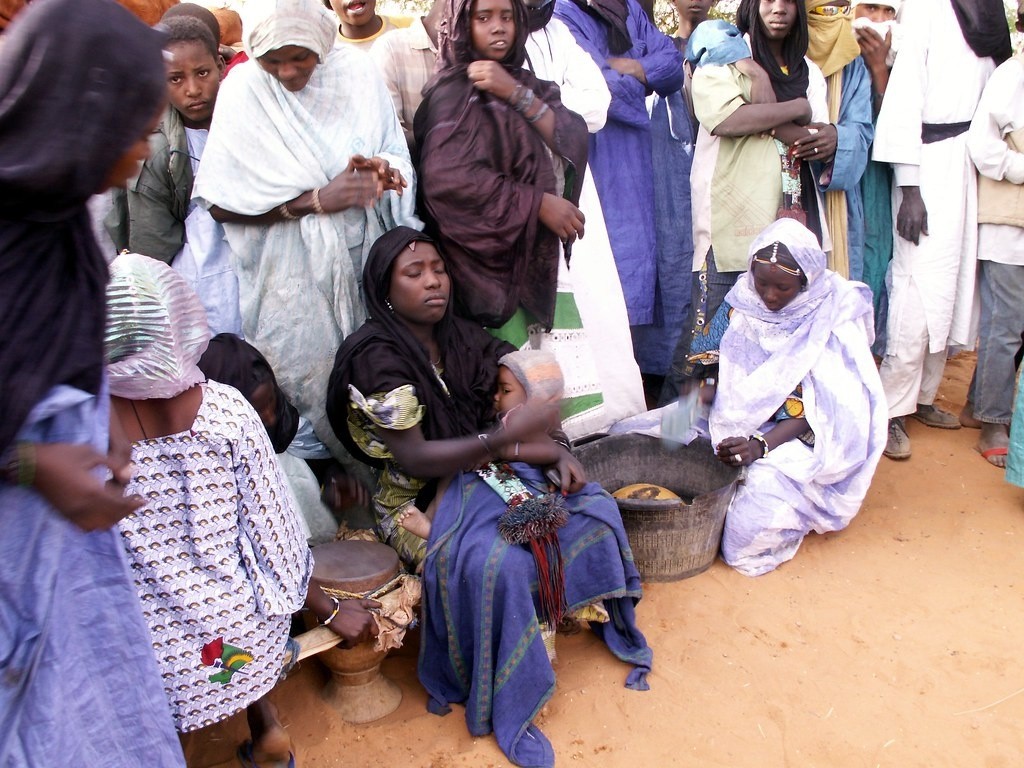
[913,405,961,429]
[960,401,983,430]
[884,420,912,460]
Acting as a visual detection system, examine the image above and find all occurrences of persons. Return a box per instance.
[554,0,1024,576]
[327,233,653,768]
[413,1,587,352]
[1,2,443,768]
[396,350,562,542]
[522,0,647,442]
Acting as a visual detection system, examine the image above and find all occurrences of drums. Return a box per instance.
[304,540,403,723]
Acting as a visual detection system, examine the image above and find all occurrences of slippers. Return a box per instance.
[235,742,296,768]
[973,439,1010,469]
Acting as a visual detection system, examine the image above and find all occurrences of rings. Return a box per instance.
[814,148,818,153]
[735,454,742,463]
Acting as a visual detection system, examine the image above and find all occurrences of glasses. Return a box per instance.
[813,6,852,14]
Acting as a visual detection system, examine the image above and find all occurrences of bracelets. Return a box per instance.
[313,186,324,213]
[509,82,535,111]
[527,103,550,123]
[317,597,340,627]
[748,434,768,458]
